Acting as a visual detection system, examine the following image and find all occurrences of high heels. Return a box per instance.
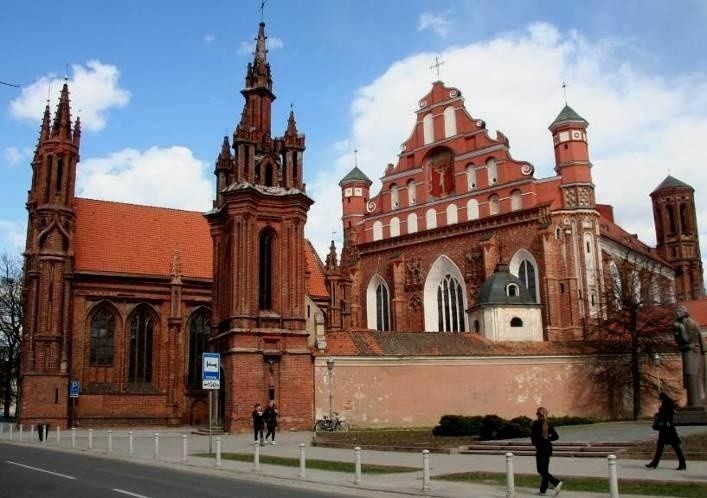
[645,462,657,469]
[676,464,686,470]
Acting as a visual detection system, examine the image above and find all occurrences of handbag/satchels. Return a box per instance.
[653,413,673,432]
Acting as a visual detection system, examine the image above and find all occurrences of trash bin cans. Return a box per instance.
[37,422,50,441]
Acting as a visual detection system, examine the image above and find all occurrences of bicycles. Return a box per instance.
[314,411,349,433]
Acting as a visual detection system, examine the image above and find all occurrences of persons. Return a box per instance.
[252,404,264,444]
[46,421,50,440]
[435,167,450,193]
[38,422,45,441]
[645,393,687,471]
[263,401,277,445]
[531,407,563,496]
[672,306,707,405]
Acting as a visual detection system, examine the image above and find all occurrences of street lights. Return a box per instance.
[654,352,662,410]
[327,356,334,432]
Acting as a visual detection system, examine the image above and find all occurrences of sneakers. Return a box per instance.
[555,481,563,496]
[254,441,275,446]
